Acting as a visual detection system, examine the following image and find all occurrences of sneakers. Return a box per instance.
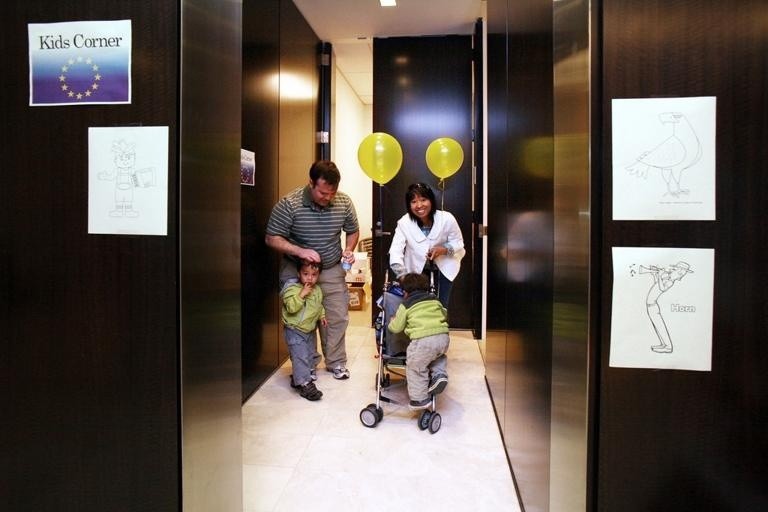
[310,368,318,381]
[408,397,432,411]
[429,373,448,395]
[301,382,323,401]
[327,366,351,379]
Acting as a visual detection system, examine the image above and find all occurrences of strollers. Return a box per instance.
[360,268,449,434]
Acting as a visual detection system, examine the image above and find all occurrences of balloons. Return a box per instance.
[356,131,403,187]
[425,138,466,180]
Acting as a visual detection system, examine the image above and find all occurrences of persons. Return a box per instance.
[388,272,450,410]
[264,160,360,380]
[278,256,328,401]
[388,182,467,309]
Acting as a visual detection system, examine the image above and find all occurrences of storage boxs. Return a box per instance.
[345,281,372,311]
[343,252,370,283]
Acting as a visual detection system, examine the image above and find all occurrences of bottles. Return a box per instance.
[341,252,354,273]
